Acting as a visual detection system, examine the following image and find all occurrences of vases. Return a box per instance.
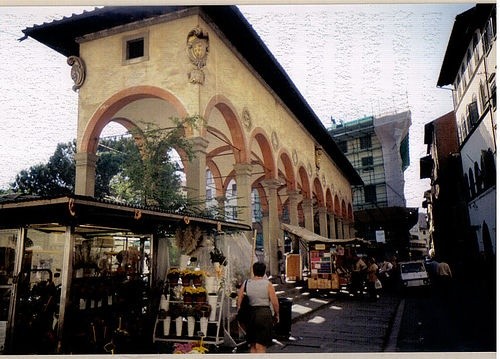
[169,278,178,282]
[196,296,206,302]
[200,317,208,337]
[182,278,190,283]
[183,297,192,302]
[193,277,201,284]
[163,319,170,336]
[205,276,217,293]
[187,316,195,336]
[207,295,218,321]
[176,317,183,336]
[160,294,170,311]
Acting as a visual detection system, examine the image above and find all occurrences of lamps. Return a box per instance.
[24,238,34,251]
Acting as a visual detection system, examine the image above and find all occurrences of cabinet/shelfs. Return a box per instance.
[152,288,224,348]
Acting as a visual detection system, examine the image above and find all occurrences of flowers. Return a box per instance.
[166,265,223,316]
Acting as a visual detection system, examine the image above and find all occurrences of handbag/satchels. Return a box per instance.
[374,279,382,289]
[239,280,249,311]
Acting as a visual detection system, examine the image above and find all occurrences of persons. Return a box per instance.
[236,261,281,353]
[350,250,399,295]
[428,257,456,296]
[436,258,453,299]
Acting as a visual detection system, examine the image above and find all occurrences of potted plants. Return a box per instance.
[76,277,113,310]
[209,251,226,272]
[85,261,97,277]
[74,260,84,278]
[229,292,238,307]
